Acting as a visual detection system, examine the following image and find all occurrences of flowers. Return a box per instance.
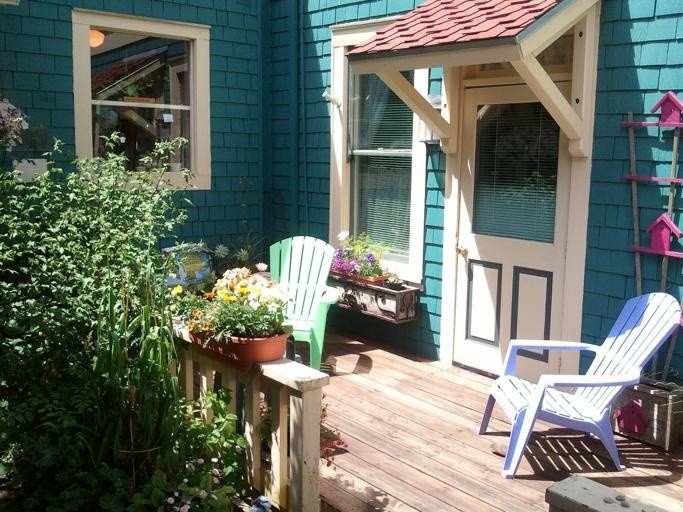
[170,263,296,349]
[330,231,384,276]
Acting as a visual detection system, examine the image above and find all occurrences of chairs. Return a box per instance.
[477,292,683,479]
[268,235,340,371]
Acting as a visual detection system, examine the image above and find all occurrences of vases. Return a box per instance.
[330,270,390,288]
[189,327,291,362]
